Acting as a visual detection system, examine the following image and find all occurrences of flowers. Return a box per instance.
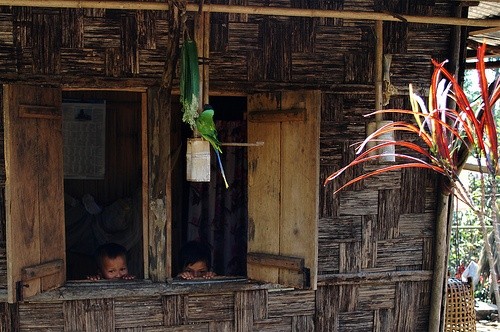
[180,89,221,149]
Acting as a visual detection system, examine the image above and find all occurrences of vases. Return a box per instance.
[184,137,212,183]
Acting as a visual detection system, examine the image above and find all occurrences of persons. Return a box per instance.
[177,241,217,281]
[87,242,138,281]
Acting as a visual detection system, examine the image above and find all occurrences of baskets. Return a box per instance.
[447,278,476,332]
